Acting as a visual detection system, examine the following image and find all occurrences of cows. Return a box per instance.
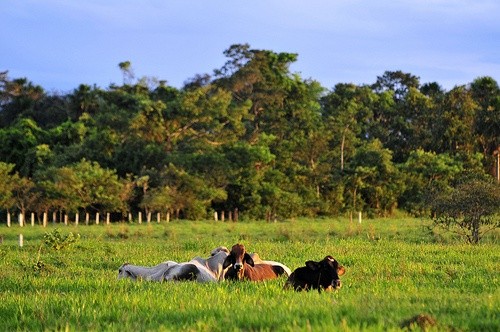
[281,256,346,292]
[222,244,284,281]
[116,260,179,281]
[250,252,293,276]
[163,246,231,282]
